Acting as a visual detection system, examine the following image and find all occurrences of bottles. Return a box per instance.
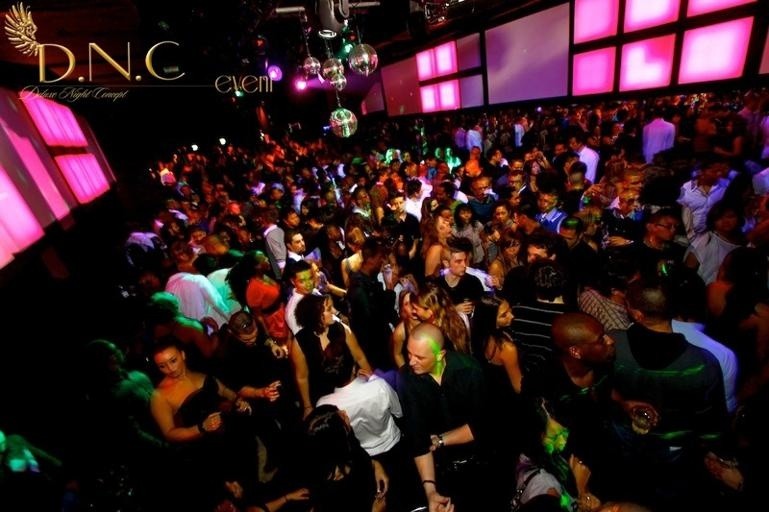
[464,298,470,305]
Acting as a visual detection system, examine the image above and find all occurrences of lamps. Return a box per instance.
[270,1,382,139]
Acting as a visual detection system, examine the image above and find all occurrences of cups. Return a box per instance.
[269,380,286,402]
[631,406,655,435]
